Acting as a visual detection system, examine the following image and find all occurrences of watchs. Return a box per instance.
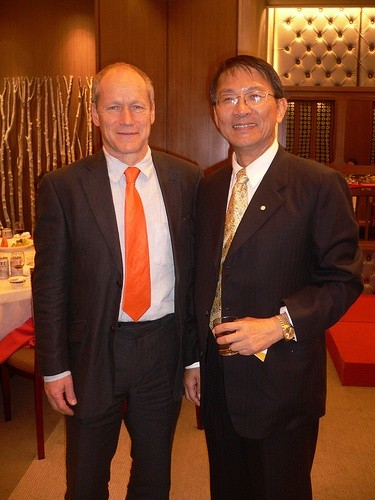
[276,315,295,342]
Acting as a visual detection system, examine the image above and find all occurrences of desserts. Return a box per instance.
[0,236,9,247]
[11,239,30,247]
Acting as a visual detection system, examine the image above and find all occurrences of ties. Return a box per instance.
[209,167,249,339]
[121,167,151,322]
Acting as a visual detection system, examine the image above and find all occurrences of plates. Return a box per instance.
[0,236,34,252]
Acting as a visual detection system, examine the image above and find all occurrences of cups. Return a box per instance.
[213,316,239,356]
[14,222,25,235]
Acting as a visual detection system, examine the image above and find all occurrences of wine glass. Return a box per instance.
[10,251,25,283]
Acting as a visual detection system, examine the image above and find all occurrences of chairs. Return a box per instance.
[343,185,375,294]
[0,268,45,460]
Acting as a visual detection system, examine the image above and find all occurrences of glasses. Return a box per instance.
[215,90,275,105]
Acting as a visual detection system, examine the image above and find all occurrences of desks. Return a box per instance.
[0,237,35,420]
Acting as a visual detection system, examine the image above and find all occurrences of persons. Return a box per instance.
[31,62,204,500]
[183,55,365,500]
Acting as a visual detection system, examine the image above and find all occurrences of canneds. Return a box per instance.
[2,228,12,239]
[0,257,9,279]
[10,257,23,276]
